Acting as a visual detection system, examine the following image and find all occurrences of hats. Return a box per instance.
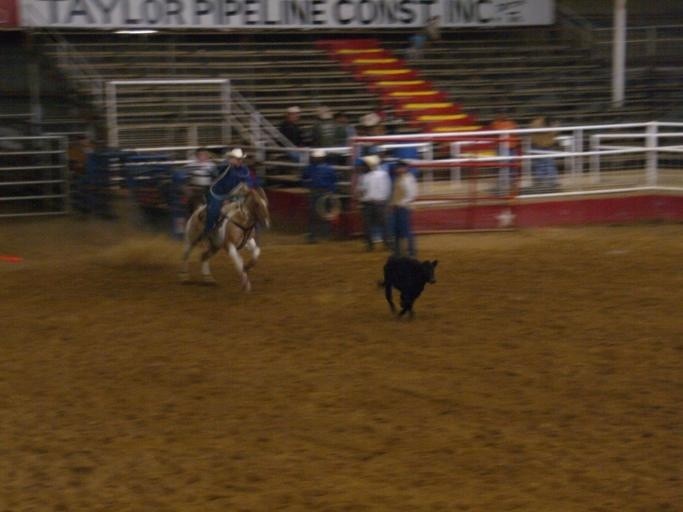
[226,149,248,159]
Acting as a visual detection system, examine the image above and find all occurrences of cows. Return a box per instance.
[376,253,438,316]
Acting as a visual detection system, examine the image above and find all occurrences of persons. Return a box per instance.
[406,13,443,60]
[487,108,558,181]
[164,148,217,238]
[70,135,97,185]
[203,149,253,231]
[302,150,338,242]
[278,106,420,180]
[356,154,417,254]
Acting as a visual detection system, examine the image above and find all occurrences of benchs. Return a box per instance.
[27,22,682,168]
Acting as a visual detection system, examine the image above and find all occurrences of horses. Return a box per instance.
[178,186,272,292]
